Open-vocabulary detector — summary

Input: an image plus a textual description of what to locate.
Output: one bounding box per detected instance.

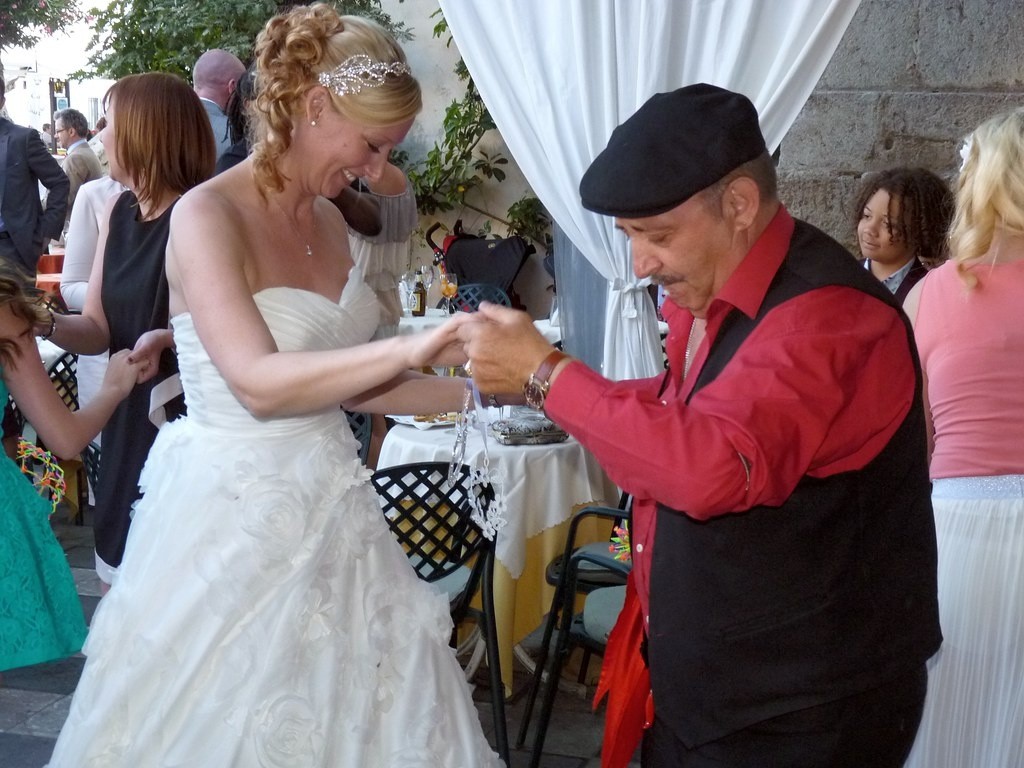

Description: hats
[580,83,768,219]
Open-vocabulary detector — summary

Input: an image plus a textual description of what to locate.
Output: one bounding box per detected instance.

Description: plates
[386,414,456,430]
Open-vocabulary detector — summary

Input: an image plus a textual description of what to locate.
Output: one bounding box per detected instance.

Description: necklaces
[269,189,316,256]
[683,318,696,379]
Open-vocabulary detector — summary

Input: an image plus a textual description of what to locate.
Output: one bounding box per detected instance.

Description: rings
[467,368,472,376]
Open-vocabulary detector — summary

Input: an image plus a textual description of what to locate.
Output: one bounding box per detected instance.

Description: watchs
[521,350,570,410]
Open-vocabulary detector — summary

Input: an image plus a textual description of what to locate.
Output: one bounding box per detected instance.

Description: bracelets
[41,310,56,340]
[462,359,472,370]
[489,393,501,408]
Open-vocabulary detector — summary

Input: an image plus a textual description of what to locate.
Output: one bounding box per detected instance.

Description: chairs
[435,284,511,314]
[366,463,511,768]
[16,352,103,527]
[514,491,632,768]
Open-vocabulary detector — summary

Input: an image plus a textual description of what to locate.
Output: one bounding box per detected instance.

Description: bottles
[412,272,426,316]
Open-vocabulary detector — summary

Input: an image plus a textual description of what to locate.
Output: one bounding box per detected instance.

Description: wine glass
[439,273,457,318]
[60,220,70,253]
[422,265,433,309]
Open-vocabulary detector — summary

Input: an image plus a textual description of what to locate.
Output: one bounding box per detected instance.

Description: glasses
[55,128,67,135]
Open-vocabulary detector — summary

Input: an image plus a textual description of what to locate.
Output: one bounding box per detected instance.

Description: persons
[50,0,512,768]
[900,107,1024,768]
[458,82,945,768]
[850,167,955,309]
[0,49,416,674]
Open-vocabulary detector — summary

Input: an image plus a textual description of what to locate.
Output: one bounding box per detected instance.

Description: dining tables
[399,308,470,376]
[375,408,633,698]
[8,336,110,506]
